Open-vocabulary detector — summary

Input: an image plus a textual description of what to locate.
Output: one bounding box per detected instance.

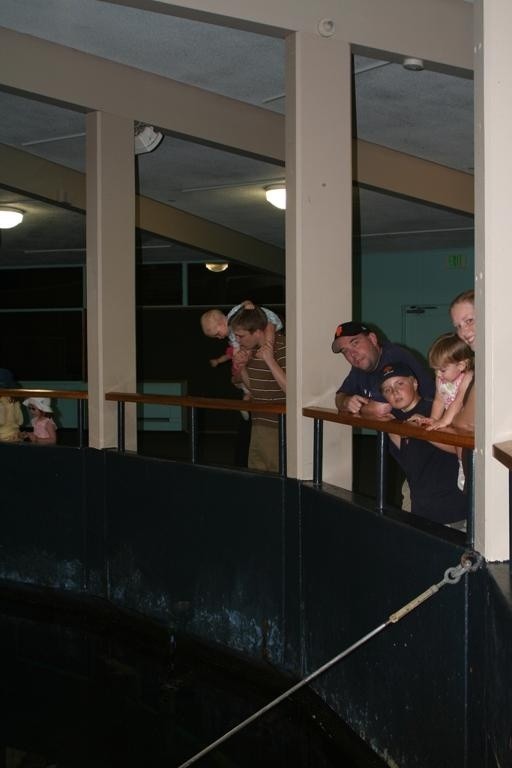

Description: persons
[200,300,283,368]
[448,288,474,351]
[404,332,474,431]
[230,306,286,472]
[331,321,436,509]
[22,397,58,444]
[377,363,468,533]
[0,368,26,443]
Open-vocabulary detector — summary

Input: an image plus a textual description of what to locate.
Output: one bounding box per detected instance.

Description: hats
[376,362,416,384]
[23,395,54,413]
[332,322,369,353]
